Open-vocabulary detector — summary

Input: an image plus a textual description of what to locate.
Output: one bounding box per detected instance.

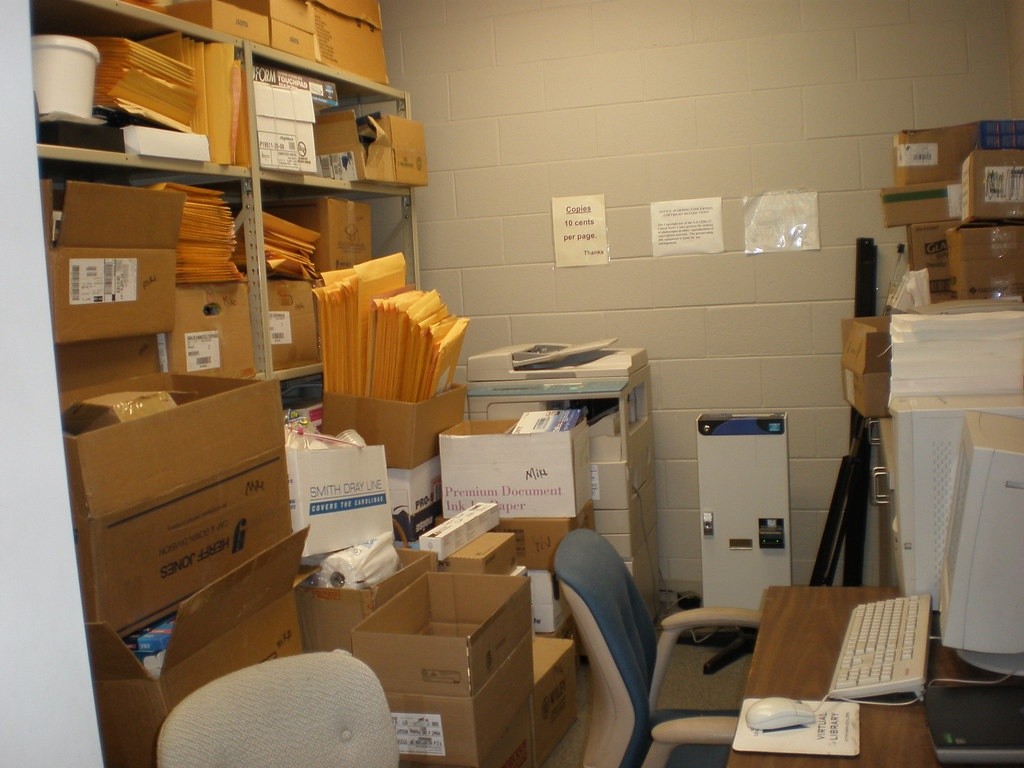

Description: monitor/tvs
[936,410,1024,677]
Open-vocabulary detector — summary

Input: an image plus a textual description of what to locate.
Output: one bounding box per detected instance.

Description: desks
[725,582,942,768]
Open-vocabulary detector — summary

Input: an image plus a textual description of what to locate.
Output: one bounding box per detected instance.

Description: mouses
[746,697,816,729]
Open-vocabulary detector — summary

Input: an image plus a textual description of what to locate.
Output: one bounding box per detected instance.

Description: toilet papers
[316,530,401,590]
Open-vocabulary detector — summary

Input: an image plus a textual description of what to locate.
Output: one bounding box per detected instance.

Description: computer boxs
[886,392,1024,611]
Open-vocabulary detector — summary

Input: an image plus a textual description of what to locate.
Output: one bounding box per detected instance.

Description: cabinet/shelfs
[30,0,421,382]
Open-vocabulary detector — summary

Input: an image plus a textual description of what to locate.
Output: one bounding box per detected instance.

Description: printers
[462,337,662,622]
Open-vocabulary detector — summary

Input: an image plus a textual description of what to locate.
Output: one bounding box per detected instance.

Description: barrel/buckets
[30,34,100,119]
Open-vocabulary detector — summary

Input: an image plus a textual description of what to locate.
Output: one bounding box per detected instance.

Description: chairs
[156,647,400,768]
[556,524,763,768]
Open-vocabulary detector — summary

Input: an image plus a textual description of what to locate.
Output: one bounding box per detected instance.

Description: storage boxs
[268,19,317,61]
[305,0,390,85]
[54,334,161,394]
[252,64,339,113]
[88,522,324,768]
[313,110,396,186]
[420,502,501,562]
[386,455,443,542]
[164,0,270,47]
[282,399,322,422]
[534,616,579,647]
[399,696,533,768]
[841,118,1024,417]
[252,81,317,174]
[437,416,591,519]
[527,569,571,634]
[321,381,468,470]
[167,280,320,377]
[352,570,535,768]
[226,195,373,279]
[311,419,322,433]
[293,547,439,658]
[375,114,429,188]
[434,498,596,571]
[529,633,579,768]
[439,531,517,576]
[57,372,293,637]
[223,0,313,34]
[36,179,187,344]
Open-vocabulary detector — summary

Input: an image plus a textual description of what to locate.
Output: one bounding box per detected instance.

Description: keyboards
[828,593,932,700]
